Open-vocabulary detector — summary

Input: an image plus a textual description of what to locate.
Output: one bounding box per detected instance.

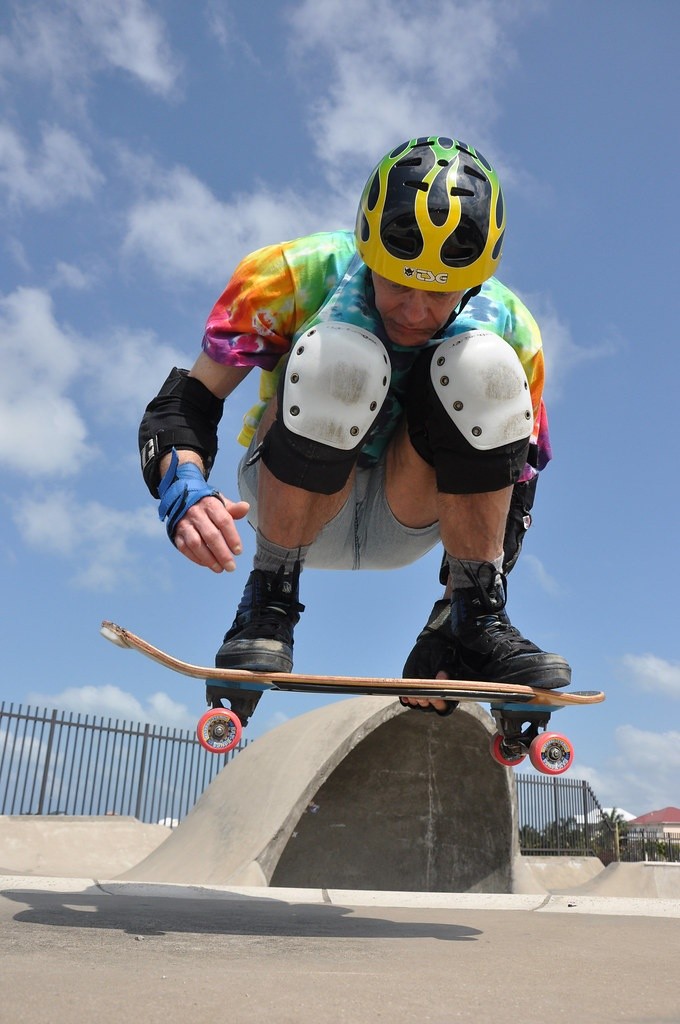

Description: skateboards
[101,620,607,774]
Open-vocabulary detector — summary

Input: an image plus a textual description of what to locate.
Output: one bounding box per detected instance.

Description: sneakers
[214,547,306,673]
[447,560,572,689]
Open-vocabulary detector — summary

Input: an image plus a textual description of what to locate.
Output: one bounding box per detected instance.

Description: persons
[138,136,571,715]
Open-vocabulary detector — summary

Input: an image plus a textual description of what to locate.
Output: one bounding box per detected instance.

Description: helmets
[354,136,506,293]
